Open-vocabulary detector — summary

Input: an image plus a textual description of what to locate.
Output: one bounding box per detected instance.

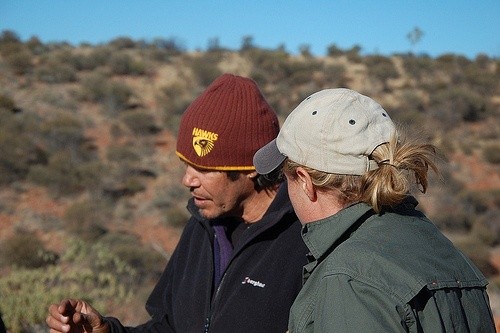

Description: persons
[42,72,307,333]
[250,86,498,333]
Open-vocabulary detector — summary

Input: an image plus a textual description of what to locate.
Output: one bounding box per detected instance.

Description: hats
[175,73,280,170]
[253,88,401,175]
